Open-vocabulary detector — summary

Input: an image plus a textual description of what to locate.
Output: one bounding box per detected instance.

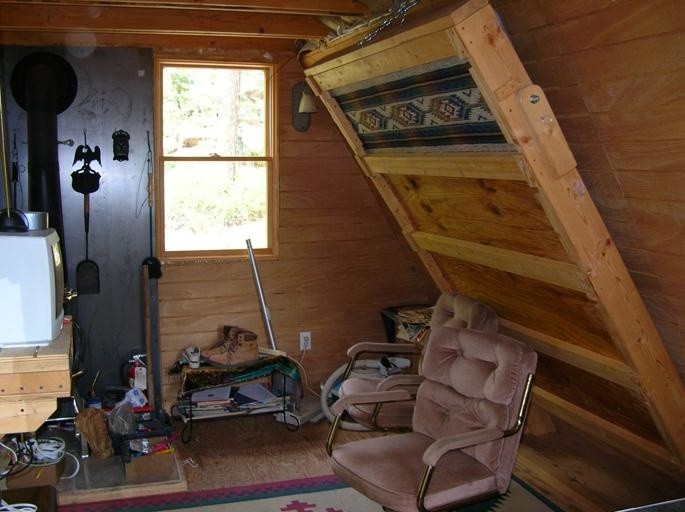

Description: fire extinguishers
[128,354,148,399]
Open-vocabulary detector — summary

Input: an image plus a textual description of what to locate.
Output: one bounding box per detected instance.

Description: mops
[243,237,336,426]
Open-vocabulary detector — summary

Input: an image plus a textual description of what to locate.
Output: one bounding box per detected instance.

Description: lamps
[292,79,319,133]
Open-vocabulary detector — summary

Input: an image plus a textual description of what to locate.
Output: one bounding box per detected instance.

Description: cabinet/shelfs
[172,354,302,443]
[0,314,76,436]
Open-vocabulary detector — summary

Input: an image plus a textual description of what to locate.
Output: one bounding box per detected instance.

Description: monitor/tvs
[1,231,64,346]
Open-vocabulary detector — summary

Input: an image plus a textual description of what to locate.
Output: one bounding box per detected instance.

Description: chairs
[321,294,499,457]
[326,326,538,511]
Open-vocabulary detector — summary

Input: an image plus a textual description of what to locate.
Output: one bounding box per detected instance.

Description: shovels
[75,193,99,295]
[142,151,161,278]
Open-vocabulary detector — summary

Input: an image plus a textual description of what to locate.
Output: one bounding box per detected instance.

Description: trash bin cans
[381,304,434,344]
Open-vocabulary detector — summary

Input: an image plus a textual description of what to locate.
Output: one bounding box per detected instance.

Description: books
[178,382,290,417]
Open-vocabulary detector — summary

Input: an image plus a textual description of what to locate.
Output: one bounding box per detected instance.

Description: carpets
[58,474,561,512]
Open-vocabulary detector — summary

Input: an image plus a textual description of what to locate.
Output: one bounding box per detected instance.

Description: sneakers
[183,325,259,369]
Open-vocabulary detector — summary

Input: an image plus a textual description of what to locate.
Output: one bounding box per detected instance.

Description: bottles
[90,399,101,409]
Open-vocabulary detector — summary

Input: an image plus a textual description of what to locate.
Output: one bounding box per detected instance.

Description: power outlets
[299,331,313,352]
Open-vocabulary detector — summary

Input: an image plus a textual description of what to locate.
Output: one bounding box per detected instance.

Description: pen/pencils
[36,462,47,479]
